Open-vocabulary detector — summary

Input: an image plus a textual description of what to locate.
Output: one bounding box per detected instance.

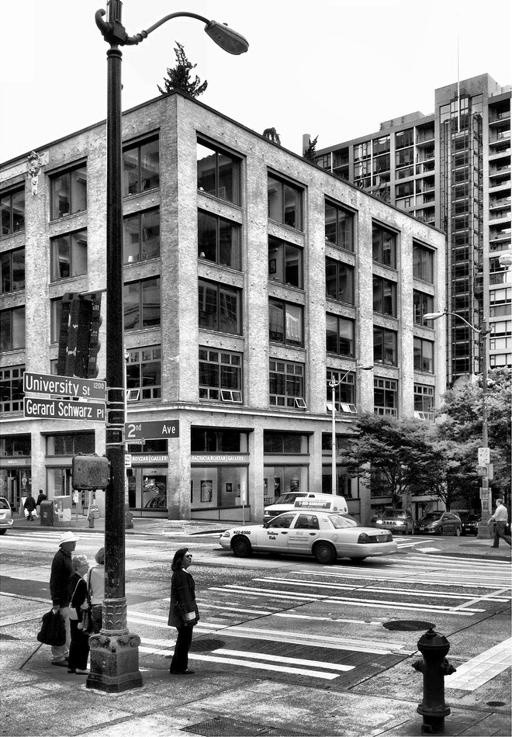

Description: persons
[36,489,49,507]
[487,498,511,549]
[164,545,203,675]
[41,532,104,679]
[22,494,36,521]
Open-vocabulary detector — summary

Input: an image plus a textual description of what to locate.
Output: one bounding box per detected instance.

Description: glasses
[184,553,193,558]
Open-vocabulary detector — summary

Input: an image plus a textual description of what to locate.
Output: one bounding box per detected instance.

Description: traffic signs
[121,418,181,441]
[21,371,104,399]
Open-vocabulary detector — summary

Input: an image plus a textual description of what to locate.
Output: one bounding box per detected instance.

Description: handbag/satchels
[82,601,103,635]
[69,606,79,621]
[37,606,68,645]
[173,602,193,620]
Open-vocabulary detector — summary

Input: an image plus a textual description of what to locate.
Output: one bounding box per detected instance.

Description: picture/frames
[200,480,212,502]
[226,483,232,492]
[290,480,299,492]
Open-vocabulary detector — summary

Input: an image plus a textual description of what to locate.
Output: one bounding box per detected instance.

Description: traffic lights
[25,398,105,422]
[95,311,105,375]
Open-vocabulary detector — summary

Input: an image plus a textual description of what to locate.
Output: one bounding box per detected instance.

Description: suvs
[0,495,17,536]
[262,491,349,530]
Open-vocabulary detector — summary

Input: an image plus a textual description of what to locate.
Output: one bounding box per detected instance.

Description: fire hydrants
[407,627,462,734]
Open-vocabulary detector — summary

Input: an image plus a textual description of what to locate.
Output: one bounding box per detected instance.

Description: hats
[59,529,83,546]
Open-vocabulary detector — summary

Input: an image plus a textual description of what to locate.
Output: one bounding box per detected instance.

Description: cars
[221,495,399,559]
[369,504,511,534]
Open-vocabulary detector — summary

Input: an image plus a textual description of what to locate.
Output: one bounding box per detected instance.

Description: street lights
[88,0,248,691]
[325,363,376,496]
[422,312,496,535]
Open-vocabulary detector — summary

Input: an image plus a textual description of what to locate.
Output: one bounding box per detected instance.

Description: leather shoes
[51,655,92,675]
[171,667,195,674]
